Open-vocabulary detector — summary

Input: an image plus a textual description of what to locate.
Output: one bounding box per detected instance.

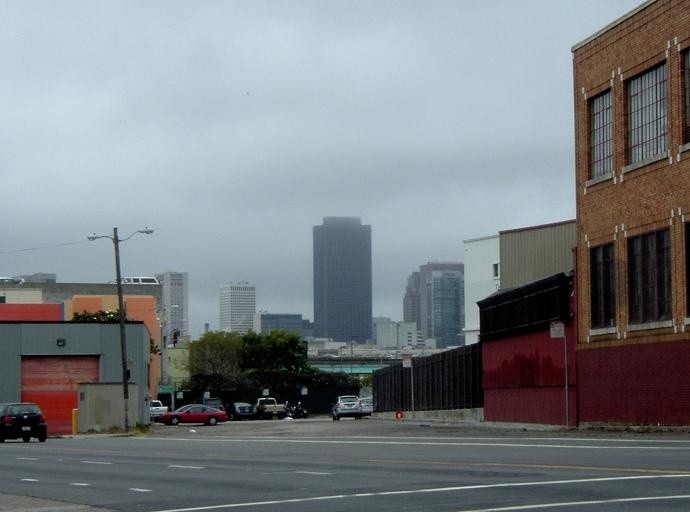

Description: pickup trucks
[252,397,285,419]
[150,400,169,421]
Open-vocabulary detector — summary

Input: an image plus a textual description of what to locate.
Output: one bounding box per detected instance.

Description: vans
[119,276,159,285]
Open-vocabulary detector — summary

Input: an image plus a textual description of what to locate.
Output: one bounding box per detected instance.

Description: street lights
[87,225,154,431]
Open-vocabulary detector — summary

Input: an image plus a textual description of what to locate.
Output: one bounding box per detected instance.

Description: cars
[332,395,363,421]
[229,402,256,420]
[160,404,228,426]
[0,403,47,444]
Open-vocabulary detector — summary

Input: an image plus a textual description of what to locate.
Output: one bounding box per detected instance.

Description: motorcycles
[286,402,308,420]
